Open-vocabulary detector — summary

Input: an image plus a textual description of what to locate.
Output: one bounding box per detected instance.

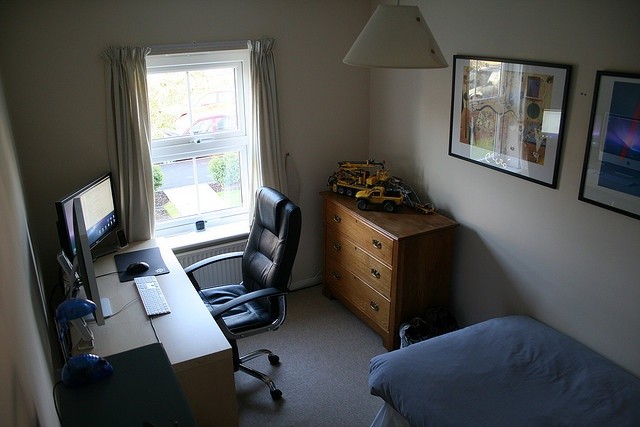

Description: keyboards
[133,276,171,316]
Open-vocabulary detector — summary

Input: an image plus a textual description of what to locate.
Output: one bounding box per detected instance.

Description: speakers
[57,252,78,282]
[71,317,94,349]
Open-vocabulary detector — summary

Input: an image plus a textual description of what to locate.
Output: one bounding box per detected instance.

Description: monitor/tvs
[67,199,112,326]
[54,172,119,262]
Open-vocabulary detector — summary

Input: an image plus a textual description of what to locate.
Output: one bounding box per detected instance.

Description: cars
[186,89,232,109]
[165,112,234,162]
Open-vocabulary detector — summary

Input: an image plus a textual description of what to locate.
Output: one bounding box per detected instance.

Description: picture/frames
[578,70,640,220]
[449,55,572,189]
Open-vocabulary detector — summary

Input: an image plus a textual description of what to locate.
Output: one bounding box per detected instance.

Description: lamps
[53,353,114,427]
[53,297,97,363]
[342,1,448,69]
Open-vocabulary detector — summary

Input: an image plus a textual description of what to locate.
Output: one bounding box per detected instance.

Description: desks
[65,238,239,427]
[55,343,199,427]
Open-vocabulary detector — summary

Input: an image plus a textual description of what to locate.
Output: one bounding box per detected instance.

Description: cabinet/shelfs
[319,190,460,352]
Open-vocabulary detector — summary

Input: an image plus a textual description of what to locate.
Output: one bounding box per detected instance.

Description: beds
[368,313,640,427]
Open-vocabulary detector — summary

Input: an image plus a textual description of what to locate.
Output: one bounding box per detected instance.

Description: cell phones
[117,229,127,247]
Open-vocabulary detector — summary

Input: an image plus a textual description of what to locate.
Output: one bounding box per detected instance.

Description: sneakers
[398,322,421,347]
[412,317,430,341]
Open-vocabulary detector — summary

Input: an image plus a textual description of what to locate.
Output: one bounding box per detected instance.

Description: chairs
[184,186,302,401]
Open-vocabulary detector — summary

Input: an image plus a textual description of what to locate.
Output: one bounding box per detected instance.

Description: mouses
[126,261,150,276]
